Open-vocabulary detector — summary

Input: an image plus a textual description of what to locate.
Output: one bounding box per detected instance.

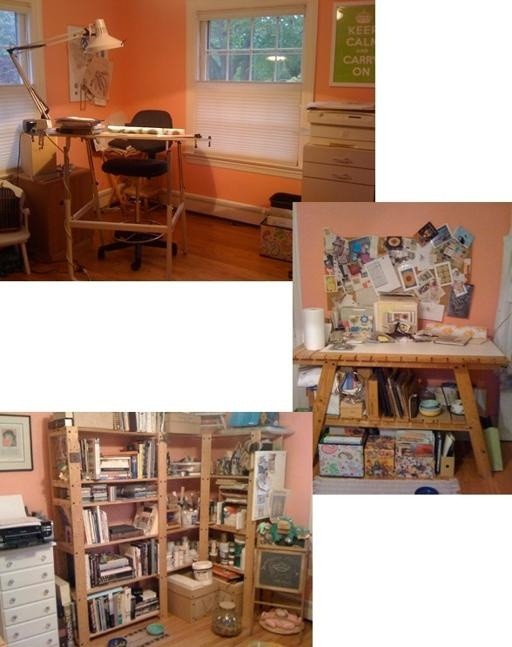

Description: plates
[108,638,126,647]
[147,624,165,635]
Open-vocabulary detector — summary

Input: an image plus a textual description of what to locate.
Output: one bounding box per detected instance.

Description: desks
[23,127,210,278]
[294,331,510,482]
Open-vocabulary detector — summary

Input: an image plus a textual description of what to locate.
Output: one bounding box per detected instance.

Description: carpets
[120,623,171,647]
[313,474,460,494]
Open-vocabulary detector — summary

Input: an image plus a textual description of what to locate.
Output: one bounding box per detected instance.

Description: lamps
[7,16,124,135]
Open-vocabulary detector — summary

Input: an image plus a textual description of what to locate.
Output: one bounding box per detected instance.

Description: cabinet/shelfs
[14,164,95,263]
[301,102,374,202]
[204,426,289,625]
[0,539,60,646]
[47,422,163,647]
[160,431,205,577]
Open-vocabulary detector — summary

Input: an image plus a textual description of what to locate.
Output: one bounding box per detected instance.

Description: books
[168,459,201,476]
[367,369,417,419]
[55,115,105,135]
[373,298,419,337]
[49,412,75,419]
[81,505,157,544]
[87,586,158,633]
[433,336,472,345]
[48,420,74,428]
[79,538,159,590]
[212,478,249,529]
[110,412,166,434]
[77,437,158,480]
[82,482,159,507]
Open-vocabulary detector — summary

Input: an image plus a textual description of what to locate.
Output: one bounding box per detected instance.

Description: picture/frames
[0,414,34,472]
[328,1,375,88]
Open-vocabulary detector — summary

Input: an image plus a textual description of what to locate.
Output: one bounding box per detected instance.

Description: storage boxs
[313,392,341,415]
[258,215,292,263]
[376,290,419,337]
[363,433,396,479]
[71,412,114,429]
[166,573,220,624]
[19,133,58,176]
[162,412,202,435]
[317,428,369,479]
[210,569,244,617]
[340,396,365,419]
[395,429,436,479]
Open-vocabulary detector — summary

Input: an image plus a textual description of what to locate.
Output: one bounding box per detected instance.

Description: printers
[0,495,55,551]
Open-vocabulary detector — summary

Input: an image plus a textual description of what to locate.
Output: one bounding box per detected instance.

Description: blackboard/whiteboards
[258,551,302,590]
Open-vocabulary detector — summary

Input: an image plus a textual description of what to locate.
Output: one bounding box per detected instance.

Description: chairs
[0,180,34,279]
[97,109,179,272]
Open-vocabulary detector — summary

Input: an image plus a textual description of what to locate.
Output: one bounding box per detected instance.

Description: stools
[121,184,162,215]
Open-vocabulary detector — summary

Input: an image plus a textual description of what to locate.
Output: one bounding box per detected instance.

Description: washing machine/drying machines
[302,306,331,351]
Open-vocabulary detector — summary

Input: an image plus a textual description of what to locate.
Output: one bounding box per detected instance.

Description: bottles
[212,602,241,637]
